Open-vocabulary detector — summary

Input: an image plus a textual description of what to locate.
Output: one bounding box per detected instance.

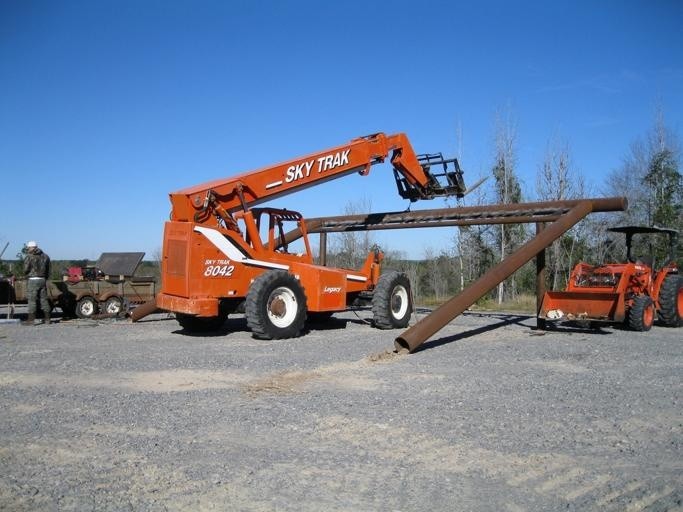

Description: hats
[27,242,37,246]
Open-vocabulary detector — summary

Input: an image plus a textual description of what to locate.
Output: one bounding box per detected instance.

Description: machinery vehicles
[538,226,683,331]
[0,253,156,318]
[155,132,487,340]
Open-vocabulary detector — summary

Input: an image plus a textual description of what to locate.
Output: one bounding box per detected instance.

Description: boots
[42,313,50,324]
[22,313,35,326]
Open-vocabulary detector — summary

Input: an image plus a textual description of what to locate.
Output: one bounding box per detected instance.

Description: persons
[21,242,51,325]
